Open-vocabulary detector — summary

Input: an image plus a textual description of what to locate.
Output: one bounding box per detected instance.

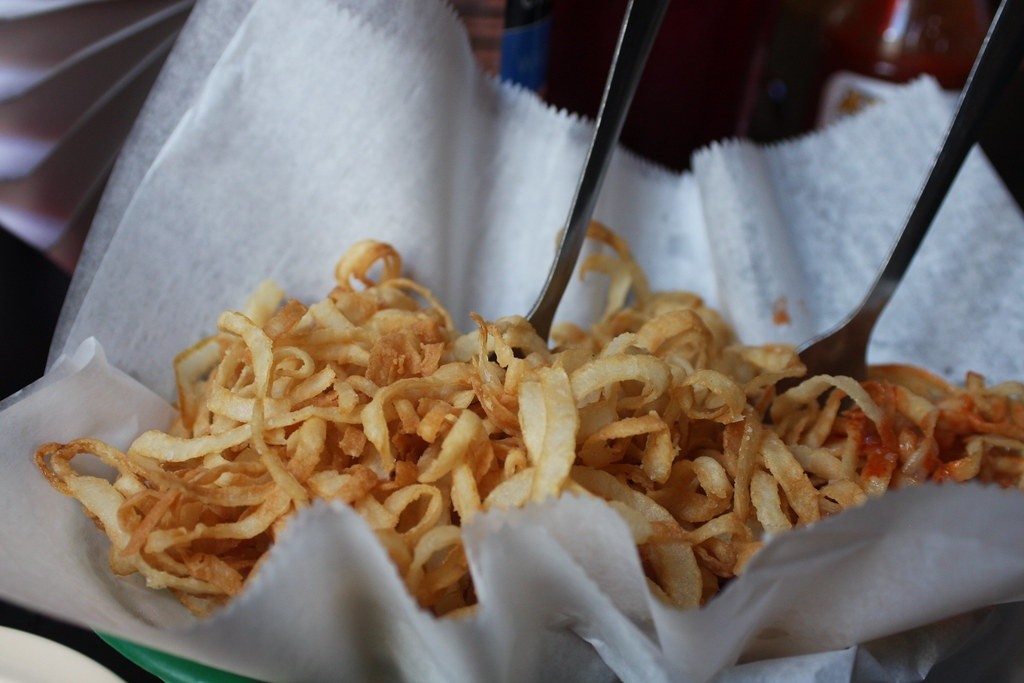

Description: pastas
[32,219,1024,617]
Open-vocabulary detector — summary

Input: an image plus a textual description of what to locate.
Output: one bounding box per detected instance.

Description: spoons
[750,0,1024,434]
[480,3,671,376]
